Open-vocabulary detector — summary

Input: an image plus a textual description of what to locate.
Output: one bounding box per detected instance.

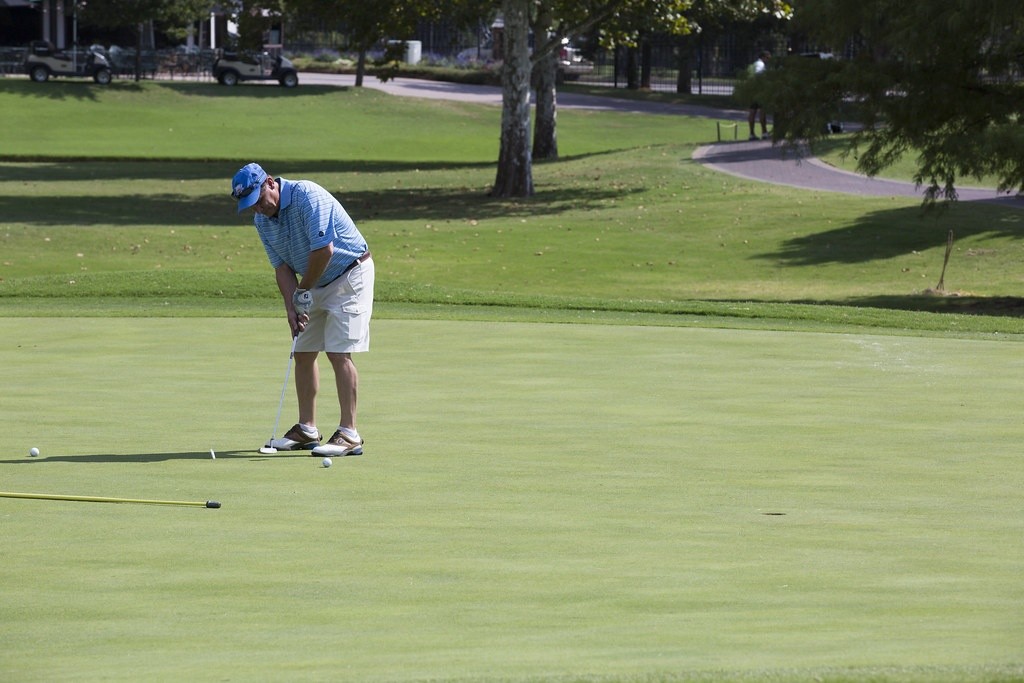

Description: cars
[488,33,594,84]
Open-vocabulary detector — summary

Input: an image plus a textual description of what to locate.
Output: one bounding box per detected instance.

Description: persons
[231,162,375,456]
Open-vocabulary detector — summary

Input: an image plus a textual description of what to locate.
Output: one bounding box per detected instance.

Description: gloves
[292,286,314,316]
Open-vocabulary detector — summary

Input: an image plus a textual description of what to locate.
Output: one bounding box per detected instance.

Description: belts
[320,249,371,288]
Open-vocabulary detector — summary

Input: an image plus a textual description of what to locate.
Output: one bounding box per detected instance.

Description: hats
[232,162,268,214]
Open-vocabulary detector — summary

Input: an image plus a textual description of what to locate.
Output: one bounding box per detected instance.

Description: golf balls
[323,458,332,467]
[30,447,39,456]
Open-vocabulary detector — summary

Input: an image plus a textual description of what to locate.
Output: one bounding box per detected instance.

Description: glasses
[230,172,265,203]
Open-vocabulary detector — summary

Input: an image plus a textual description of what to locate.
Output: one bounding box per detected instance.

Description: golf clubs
[259,314,300,454]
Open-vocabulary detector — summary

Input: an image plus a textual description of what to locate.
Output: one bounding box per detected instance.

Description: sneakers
[266,423,322,451]
[311,429,364,457]
